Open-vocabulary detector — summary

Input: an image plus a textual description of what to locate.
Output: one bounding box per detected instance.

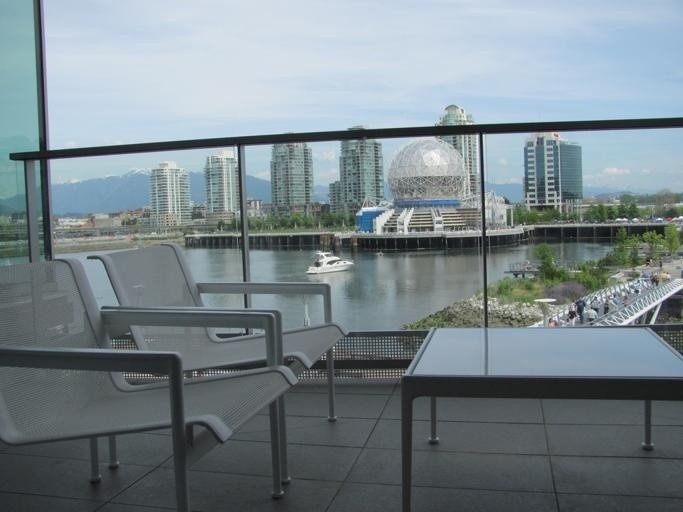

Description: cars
[612,216,683,224]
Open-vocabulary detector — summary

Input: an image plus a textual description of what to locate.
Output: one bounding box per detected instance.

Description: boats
[305,249,354,275]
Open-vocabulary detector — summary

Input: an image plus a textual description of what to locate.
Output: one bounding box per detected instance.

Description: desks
[402,326,683,511]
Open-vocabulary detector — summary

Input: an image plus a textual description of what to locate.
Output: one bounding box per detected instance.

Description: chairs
[0,259,286,512]
[87,244,346,485]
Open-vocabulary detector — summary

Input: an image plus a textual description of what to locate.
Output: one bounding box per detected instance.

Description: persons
[547,256,670,328]
